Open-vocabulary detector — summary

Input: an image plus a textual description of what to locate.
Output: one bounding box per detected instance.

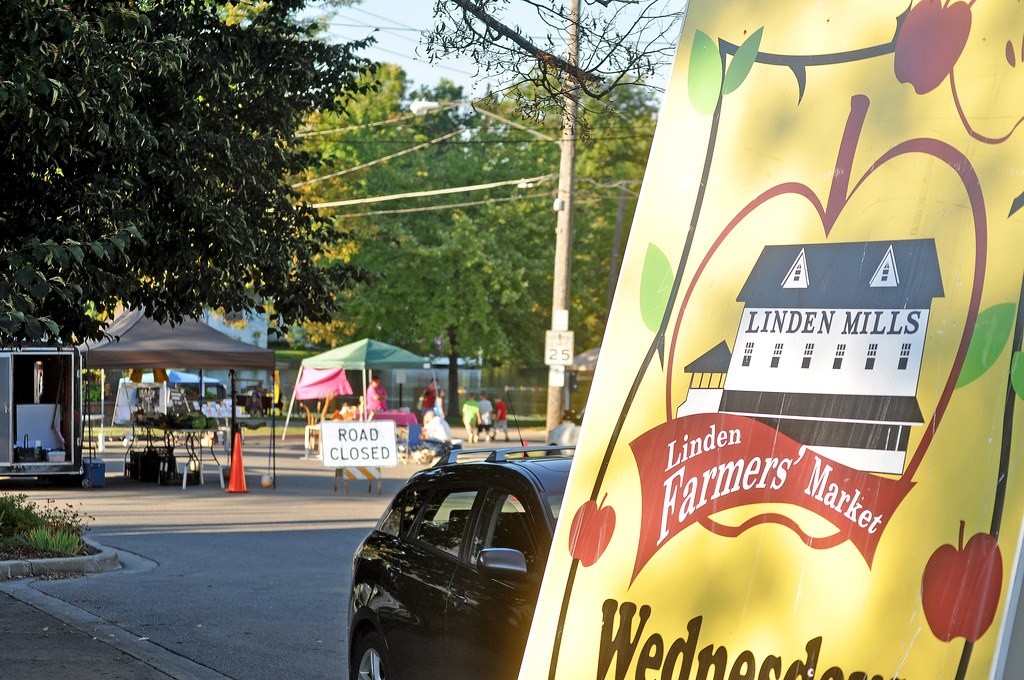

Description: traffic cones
[225,432,249,493]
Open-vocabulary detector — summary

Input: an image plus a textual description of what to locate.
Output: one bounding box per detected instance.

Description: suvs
[347,446,578,680]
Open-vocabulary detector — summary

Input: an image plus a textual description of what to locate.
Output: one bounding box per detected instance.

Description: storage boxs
[47,452,66,462]
[125,446,200,486]
[82,457,105,487]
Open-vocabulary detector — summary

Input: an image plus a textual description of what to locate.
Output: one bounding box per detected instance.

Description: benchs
[447,509,537,555]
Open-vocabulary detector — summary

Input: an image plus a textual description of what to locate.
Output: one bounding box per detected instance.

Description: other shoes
[485,435,496,443]
[469,434,479,443]
[505,436,510,442]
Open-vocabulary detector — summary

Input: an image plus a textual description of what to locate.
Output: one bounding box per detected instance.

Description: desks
[356,411,418,442]
[304,425,322,461]
[122,424,225,490]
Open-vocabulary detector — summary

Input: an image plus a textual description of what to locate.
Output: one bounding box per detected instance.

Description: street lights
[408,0,582,456]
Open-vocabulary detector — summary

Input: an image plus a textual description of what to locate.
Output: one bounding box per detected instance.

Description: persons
[419,380,452,443]
[462,391,509,443]
[365,376,388,413]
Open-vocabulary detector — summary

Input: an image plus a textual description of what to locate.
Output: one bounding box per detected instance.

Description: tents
[283,338,438,441]
[75,300,277,491]
[565,348,600,410]
